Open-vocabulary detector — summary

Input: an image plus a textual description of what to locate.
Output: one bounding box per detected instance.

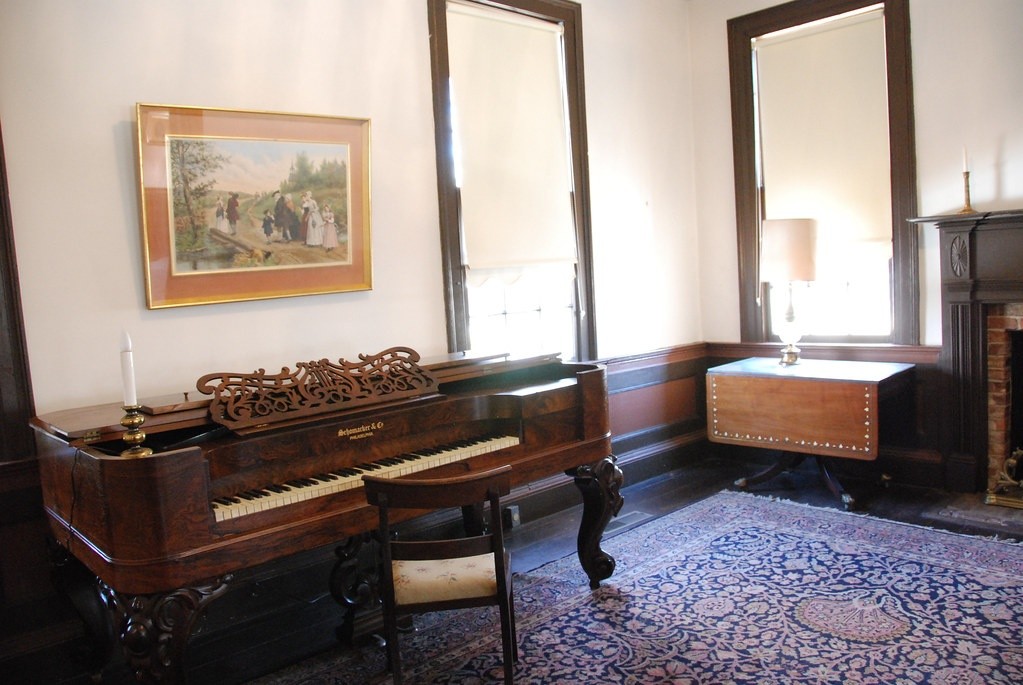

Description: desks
[706,354,917,511]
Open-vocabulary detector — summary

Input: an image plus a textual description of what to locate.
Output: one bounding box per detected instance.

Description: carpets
[244,487,1023,685]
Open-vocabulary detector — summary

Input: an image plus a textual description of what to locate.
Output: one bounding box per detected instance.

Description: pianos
[23,346,623,680]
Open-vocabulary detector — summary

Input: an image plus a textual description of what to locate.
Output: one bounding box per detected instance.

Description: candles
[119,330,138,407]
[961,143,968,171]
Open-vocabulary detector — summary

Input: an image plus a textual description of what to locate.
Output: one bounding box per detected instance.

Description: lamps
[758,218,818,366]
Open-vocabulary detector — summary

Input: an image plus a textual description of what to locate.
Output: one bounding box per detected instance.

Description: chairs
[361,463,519,685]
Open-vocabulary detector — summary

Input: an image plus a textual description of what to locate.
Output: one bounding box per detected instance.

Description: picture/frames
[130,102,374,310]
